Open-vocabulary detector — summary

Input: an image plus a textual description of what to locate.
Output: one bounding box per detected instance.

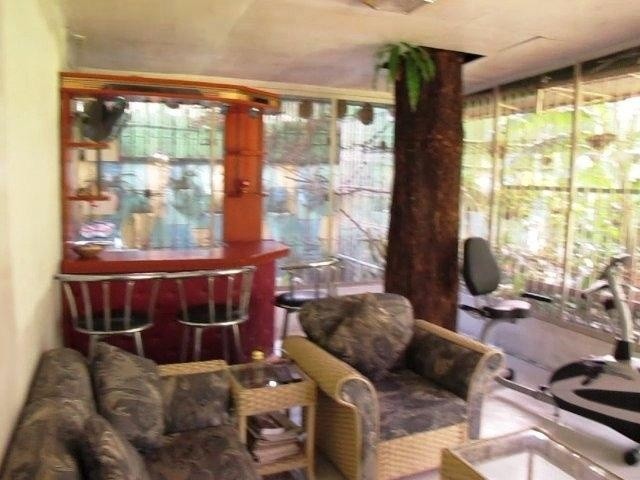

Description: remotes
[284,364,304,380]
[273,367,288,383]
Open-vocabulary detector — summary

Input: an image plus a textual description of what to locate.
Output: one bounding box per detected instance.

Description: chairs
[53,271,165,358]
[268,255,339,342]
[282,319,506,480]
[165,265,257,366]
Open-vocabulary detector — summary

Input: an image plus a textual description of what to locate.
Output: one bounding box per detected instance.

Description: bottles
[251,350,264,385]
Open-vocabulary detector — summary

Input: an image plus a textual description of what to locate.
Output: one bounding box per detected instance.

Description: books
[246,410,304,464]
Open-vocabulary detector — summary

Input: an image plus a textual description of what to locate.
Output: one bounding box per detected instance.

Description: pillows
[0,342,232,479]
[299,293,414,383]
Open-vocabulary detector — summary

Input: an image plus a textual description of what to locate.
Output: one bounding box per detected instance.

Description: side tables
[228,358,316,480]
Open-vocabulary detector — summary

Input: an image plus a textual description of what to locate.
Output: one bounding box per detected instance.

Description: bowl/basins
[71,245,105,257]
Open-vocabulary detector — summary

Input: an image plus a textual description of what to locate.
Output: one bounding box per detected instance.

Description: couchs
[1,349,262,480]
